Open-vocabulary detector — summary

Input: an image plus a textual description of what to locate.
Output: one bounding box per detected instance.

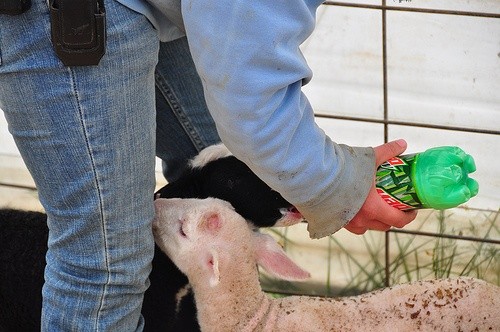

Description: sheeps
[151,197,499,332]
[0,143,306,332]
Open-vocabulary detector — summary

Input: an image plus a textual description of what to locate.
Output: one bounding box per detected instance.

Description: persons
[0,0,422,332]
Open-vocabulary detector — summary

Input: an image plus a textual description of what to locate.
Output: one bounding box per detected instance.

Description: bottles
[375,146,479,212]
